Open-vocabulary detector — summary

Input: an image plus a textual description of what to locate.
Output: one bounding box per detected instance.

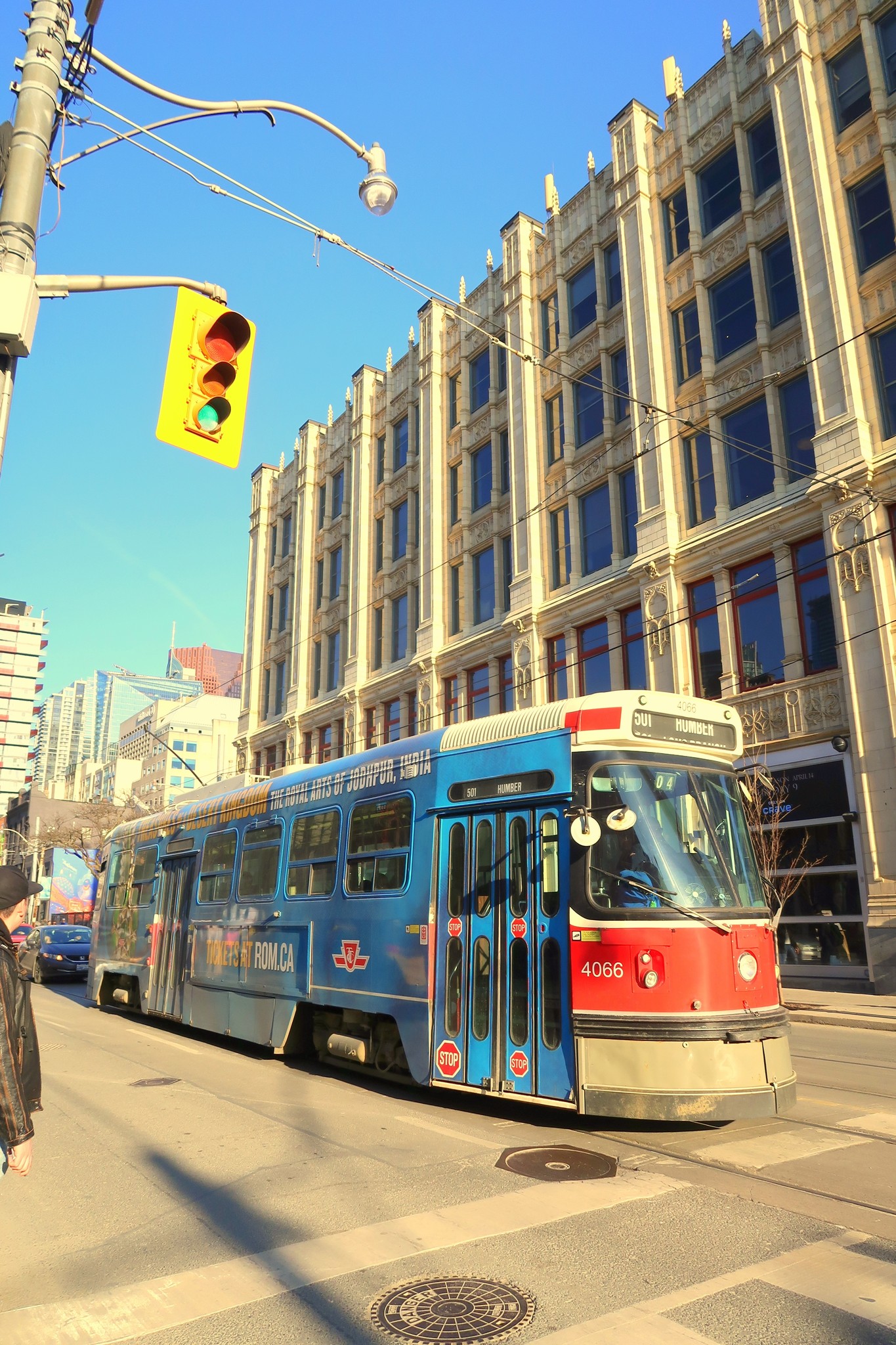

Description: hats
[0,865,43,909]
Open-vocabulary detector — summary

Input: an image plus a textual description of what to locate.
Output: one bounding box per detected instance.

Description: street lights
[1,828,39,924]
[2,0,399,480]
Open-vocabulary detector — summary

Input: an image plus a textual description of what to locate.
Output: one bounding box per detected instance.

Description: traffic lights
[156,286,256,470]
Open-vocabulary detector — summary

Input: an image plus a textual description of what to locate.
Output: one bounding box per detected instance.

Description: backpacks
[617,870,660,908]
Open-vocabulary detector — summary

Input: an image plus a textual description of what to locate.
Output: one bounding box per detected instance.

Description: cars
[10,924,34,949]
[17,924,92,984]
[785,931,833,963]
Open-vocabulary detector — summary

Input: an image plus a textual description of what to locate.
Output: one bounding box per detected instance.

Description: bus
[89,690,797,1121]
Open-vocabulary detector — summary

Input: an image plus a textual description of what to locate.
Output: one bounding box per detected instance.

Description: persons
[69,932,82,942]
[624,817,672,877]
[45,931,58,943]
[0,866,43,1176]
[779,924,800,965]
[40,917,47,924]
[59,918,68,925]
[47,920,57,925]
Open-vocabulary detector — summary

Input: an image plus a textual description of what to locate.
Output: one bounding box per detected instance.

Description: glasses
[649,828,663,833]
